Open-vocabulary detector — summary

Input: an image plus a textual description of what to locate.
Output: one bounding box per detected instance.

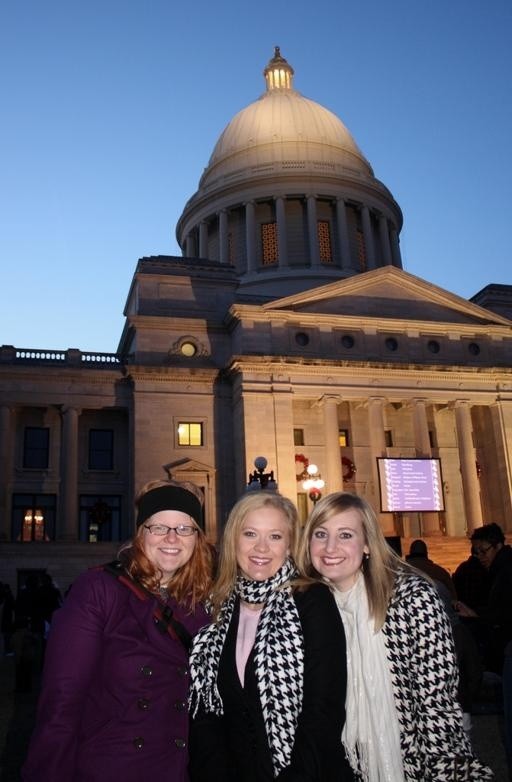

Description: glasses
[144,525,198,536]
[471,544,493,555]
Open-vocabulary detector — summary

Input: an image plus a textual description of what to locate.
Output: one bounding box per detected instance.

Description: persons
[17,480,211,781]
[189,491,356,781]
[404,522,512,782]
[0,568,72,673]
[294,489,495,782]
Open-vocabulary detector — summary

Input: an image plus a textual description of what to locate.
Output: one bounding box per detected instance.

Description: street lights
[302,463,325,508]
[248,456,275,486]
